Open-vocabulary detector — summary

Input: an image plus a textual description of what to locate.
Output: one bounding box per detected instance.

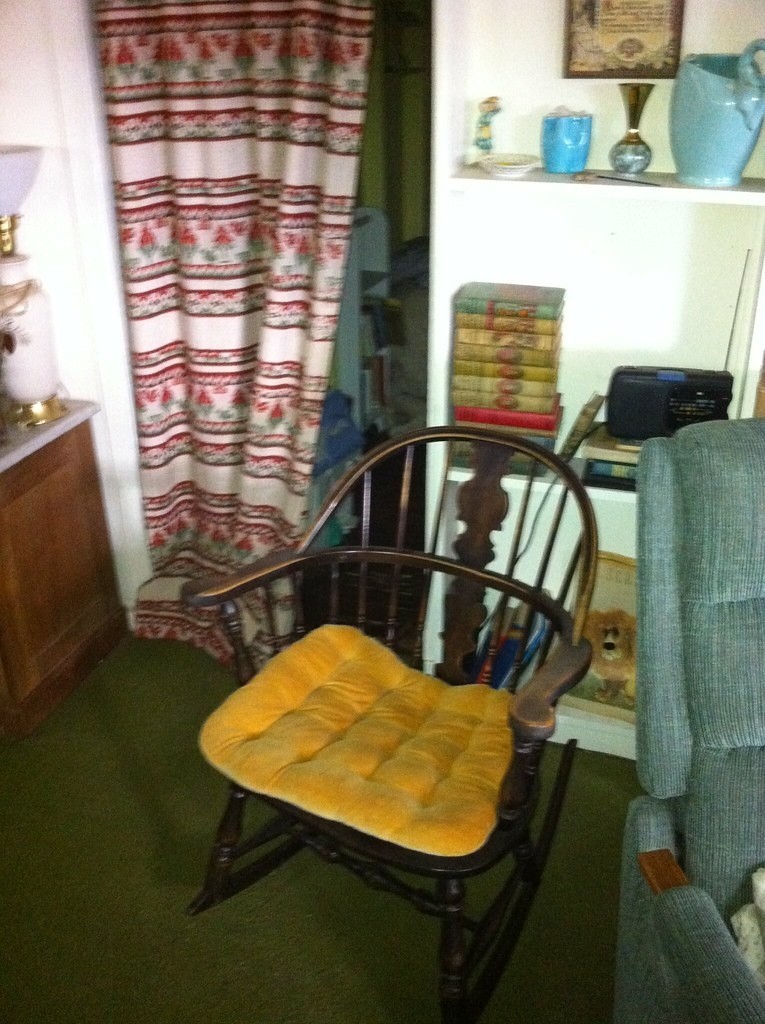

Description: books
[558,391,605,466]
[582,418,642,479]
[449,282,566,479]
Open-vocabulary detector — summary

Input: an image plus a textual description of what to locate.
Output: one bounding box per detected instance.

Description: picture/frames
[559,549,639,721]
[563,0,686,79]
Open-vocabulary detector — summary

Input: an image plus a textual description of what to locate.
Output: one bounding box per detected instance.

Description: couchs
[611,413,765,1023]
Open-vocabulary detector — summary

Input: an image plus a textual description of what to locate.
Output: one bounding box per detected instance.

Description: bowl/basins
[477,152,539,179]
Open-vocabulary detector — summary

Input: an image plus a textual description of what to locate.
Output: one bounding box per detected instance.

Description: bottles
[607,82,655,172]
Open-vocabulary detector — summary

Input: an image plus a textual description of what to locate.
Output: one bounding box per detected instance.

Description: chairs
[183,429,606,1023]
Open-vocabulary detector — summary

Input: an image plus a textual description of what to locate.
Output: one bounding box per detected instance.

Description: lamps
[0,143,67,430]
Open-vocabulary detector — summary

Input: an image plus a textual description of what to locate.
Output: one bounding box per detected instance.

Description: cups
[540,115,593,173]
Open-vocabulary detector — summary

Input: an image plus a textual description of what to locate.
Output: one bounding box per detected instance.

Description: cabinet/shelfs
[423,0,765,763]
[0,419,127,740]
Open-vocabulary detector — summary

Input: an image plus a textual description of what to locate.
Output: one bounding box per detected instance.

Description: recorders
[606,365,735,441]
